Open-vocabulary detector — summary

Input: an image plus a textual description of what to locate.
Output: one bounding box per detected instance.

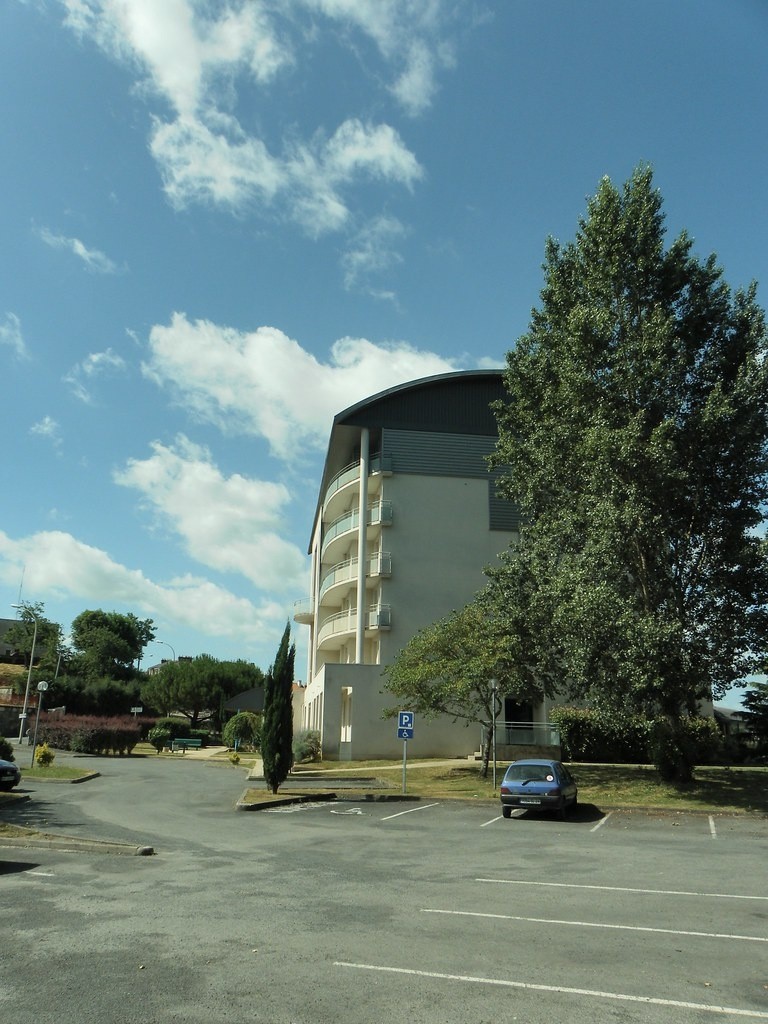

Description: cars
[500,759,577,818]
[0,759,21,792]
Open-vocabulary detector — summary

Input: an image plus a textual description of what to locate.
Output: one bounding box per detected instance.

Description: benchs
[175,738,201,750]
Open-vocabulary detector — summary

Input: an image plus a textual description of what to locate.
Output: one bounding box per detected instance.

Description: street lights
[156,640,176,662]
[488,678,501,790]
[10,604,37,744]
[30,681,48,768]
